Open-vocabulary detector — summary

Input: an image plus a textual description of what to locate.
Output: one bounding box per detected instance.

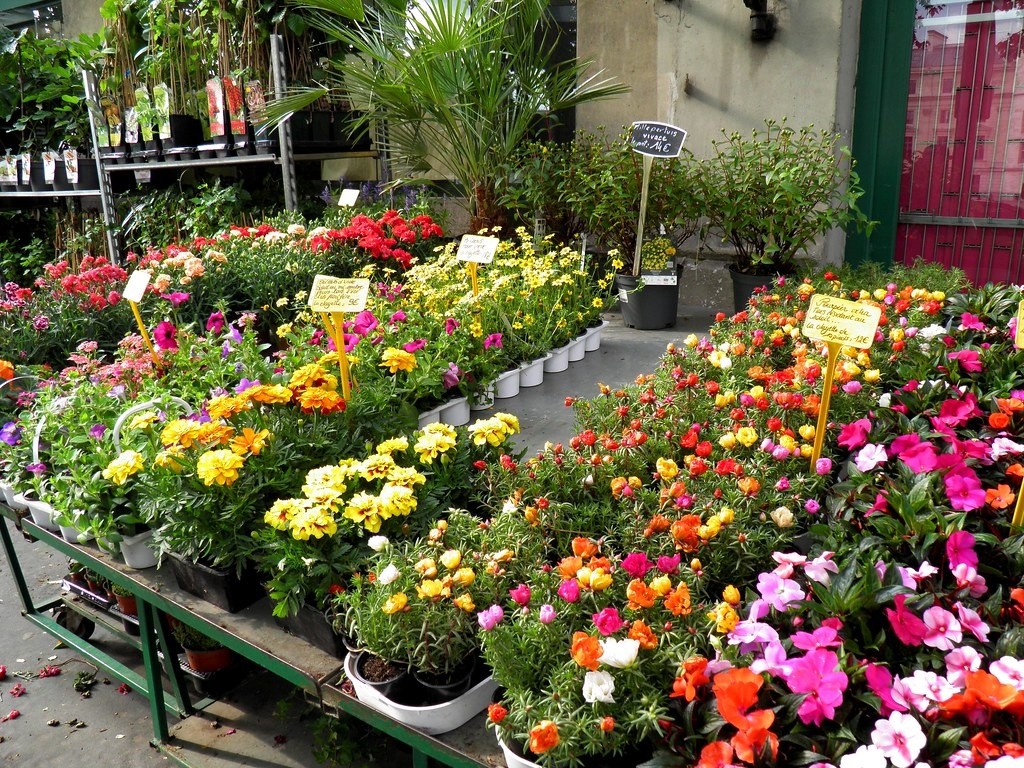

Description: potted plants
[687,116,882,316]
[0,0,410,192]
[555,124,725,334]
[502,129,637,299]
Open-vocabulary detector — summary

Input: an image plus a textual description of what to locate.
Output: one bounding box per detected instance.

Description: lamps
[744,0,777,42]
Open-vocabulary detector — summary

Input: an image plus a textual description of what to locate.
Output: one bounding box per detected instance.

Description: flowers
[0,209,1024,768]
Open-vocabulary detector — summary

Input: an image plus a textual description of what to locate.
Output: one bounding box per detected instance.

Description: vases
[491,686,515,711]
[12,492,60,532]
[0,479,28,510]
[411,652,476,705]
[95,537,122,554]
[494,725,542,768]
[354,650,423,707]
[344,651,500,736]
[160,541,272,614]
[473,352,554,411]
[542,320,610,372]
[119,529,167,569]
[591,734,657,768]
[53,516,93,543]
[536,752,594,768]
[342,634,361,658]
[417,391,479,431]
[270,595,346,658]
